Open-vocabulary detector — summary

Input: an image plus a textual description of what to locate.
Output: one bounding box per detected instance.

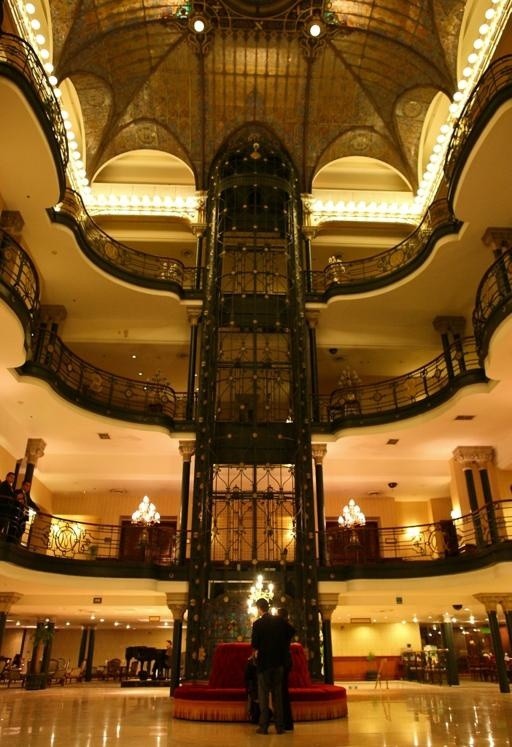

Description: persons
[250,598,285,734]
[0,471,24,541]
[9,481,42,543]
[277,608,296,734]
[152,639,172,680]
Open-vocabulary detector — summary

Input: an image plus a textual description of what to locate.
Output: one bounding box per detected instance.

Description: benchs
[170,640,348,721]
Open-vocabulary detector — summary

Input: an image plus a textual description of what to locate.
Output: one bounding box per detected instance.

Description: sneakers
[255,727,268,734]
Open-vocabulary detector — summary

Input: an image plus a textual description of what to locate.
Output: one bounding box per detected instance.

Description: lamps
[306,14,323,36]
[245,574,278,629]
[131,494,161,544]
[337,497,366,548]
[190,11,208,35]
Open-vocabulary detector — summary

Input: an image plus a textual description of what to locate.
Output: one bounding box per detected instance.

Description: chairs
[466,654,497,682]
[165,650,187,680]
[0,656,87,688]
